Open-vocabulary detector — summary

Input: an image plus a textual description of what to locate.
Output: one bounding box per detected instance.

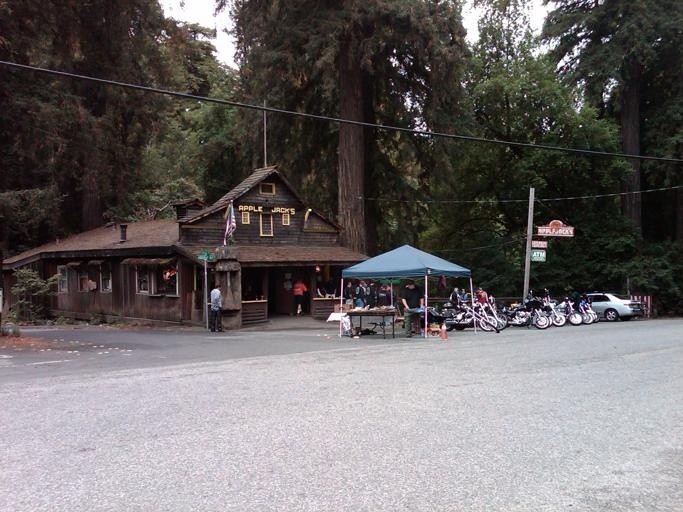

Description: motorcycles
[440,287,598,332]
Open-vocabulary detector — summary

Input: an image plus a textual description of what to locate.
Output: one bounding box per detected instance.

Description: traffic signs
[197,255,206,260]
[207,254,217,261]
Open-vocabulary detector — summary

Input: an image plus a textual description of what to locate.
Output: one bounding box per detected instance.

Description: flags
[223,204,237,247]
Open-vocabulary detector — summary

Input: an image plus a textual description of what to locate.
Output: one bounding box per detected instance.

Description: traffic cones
[440,320,447,339]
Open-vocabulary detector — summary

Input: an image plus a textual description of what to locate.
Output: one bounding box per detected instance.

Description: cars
[585,292,643,322]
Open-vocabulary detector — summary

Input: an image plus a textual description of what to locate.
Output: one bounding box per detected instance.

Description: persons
[341,274,593,336]
[210,283,226,332]
[292,277,308,319]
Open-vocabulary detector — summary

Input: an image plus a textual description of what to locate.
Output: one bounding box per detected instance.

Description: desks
[347,307,397,340]
